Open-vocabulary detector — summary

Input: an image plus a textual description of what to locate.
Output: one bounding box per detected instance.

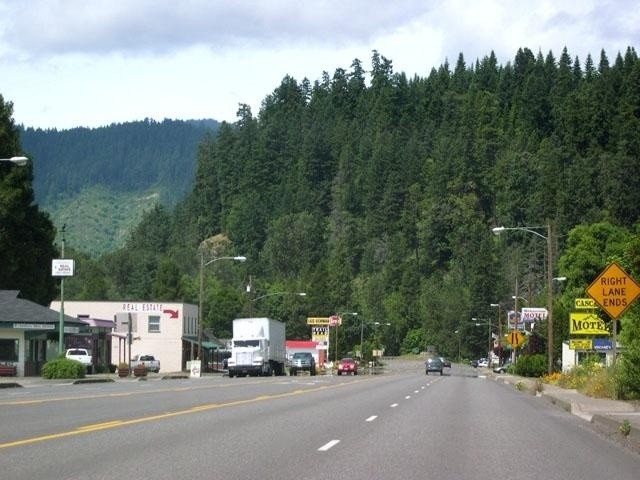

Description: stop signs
[330,315,342,327]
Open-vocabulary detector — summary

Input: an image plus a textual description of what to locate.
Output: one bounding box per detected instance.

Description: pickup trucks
[65,347,94,375]
[131,354,160,373]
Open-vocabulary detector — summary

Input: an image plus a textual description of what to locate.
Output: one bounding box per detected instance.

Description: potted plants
[134,364,149,376]
[118,362,131,377]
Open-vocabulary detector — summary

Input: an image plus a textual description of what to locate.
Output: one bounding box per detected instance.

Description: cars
[438,356,451,368]
[492,361,511,374]
[425,358,443,376]
[337,358,358,375]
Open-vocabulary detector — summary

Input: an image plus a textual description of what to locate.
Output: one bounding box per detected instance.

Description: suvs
[289,352,316,376]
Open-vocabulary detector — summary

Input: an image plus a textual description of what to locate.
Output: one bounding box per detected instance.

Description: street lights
[490,303,502,366]
[197,256,247,360]
[247,275,307,317]
[335,312,358,367]
[454,330,461,366]
[374,323,391,363]
[359,320,380,361]
[472,317,491,366]
[491,223,553,375]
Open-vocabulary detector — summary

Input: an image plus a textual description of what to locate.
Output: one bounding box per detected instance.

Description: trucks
[227,317,286,377]
